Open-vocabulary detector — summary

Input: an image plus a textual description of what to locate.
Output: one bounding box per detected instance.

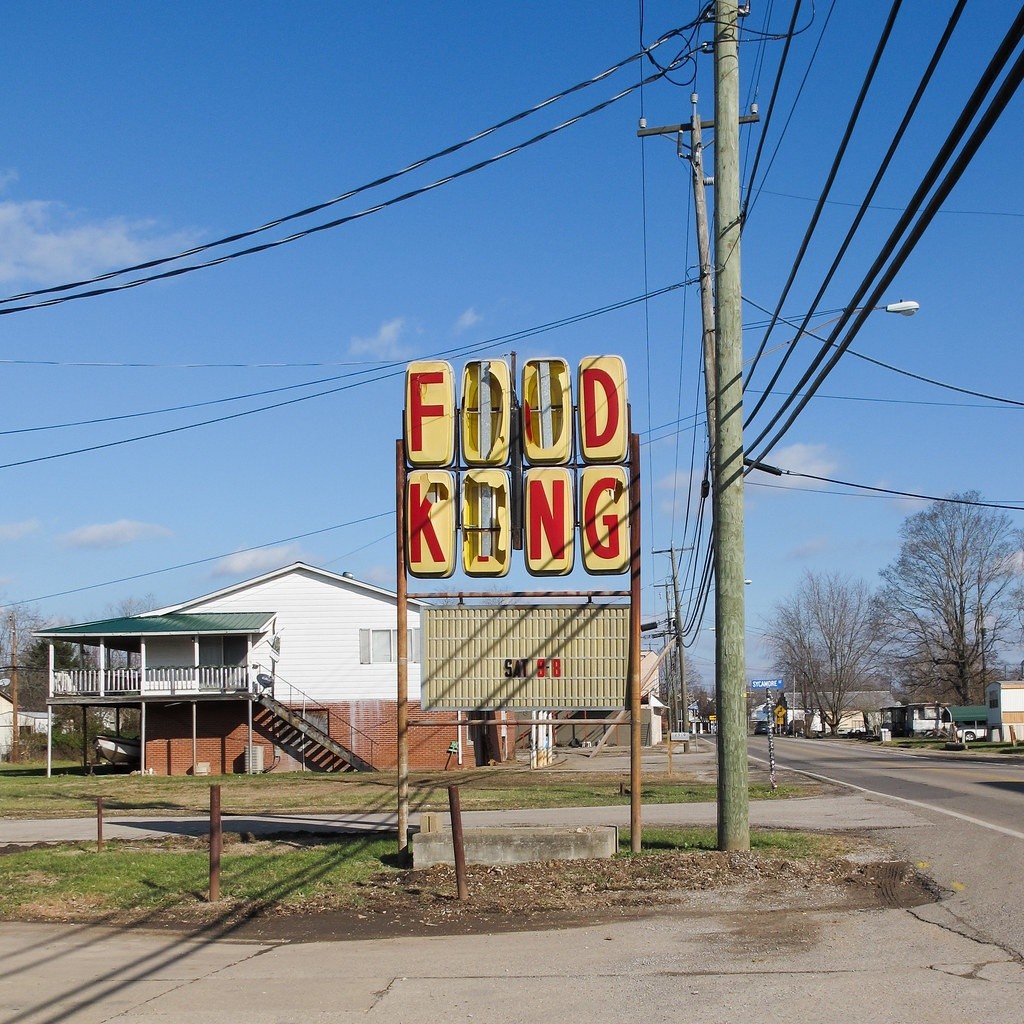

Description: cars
[956,720,988,742]
[850,723,875,735]
[754,721,768,735]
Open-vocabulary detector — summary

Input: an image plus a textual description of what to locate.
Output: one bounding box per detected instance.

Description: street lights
[703,302,917,496]
[673,580,754,753]
